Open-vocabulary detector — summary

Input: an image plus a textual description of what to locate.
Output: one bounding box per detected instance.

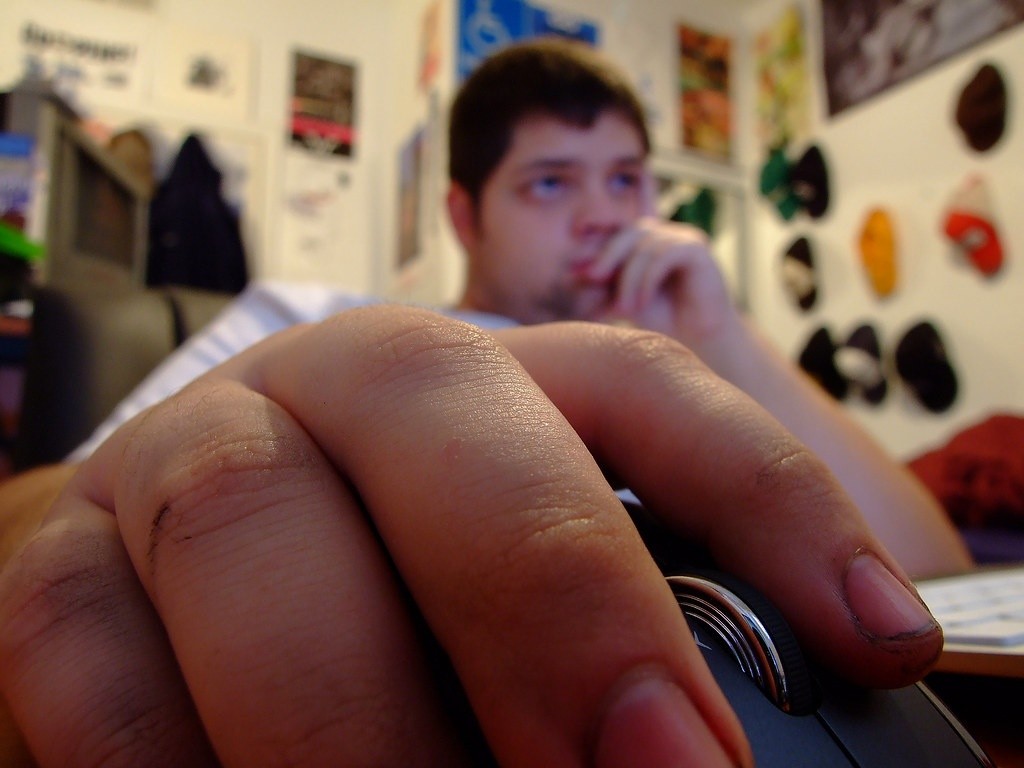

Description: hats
[780,238,817,308]
[858,209,899,296]
[934,163,1006,274]
[893,321,958,413]
[669,187,715,236]
[759,148,801,220]
[791,146,828,217]
[833,323,888,404]
[797,328,852,400]
[956,63,1008,152]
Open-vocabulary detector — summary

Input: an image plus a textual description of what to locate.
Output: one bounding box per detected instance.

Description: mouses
[384,494,995,768]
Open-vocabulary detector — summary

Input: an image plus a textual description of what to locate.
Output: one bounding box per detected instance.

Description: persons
[0,37,973,768]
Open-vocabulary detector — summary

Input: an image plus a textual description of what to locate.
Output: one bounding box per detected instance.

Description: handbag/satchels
[145,133,250,293]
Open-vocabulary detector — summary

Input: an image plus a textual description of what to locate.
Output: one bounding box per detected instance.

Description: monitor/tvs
[27,108,146,300]
